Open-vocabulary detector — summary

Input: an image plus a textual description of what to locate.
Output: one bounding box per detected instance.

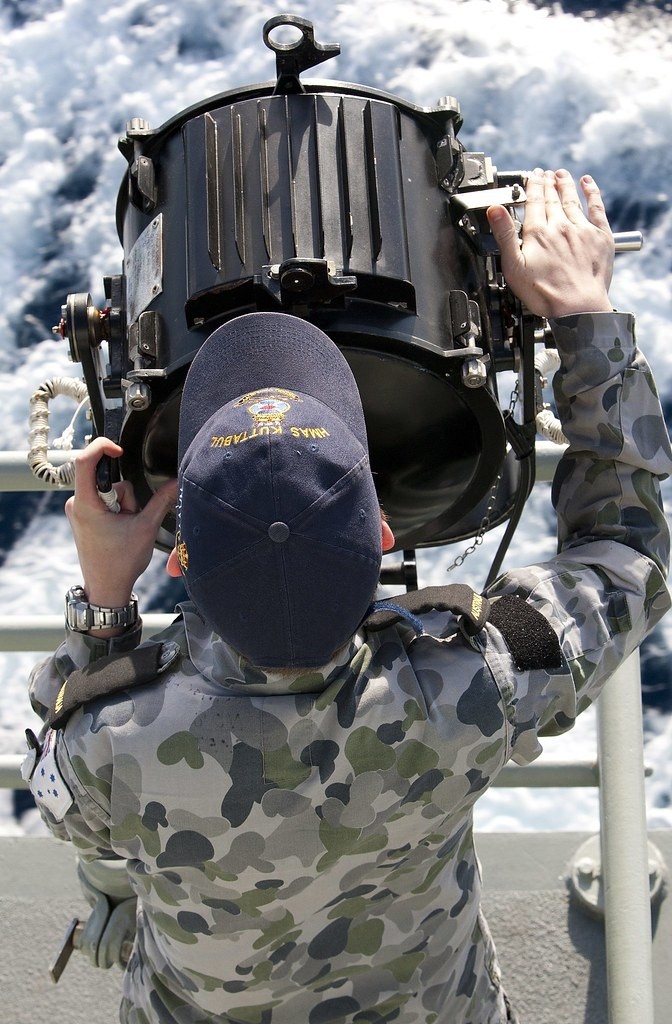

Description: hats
[174,311,384,667]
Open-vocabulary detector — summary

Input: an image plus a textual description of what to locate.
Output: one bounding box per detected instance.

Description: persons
[21,167,672,1024]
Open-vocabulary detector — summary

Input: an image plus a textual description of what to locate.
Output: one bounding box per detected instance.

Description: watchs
[63,584,143,637]
